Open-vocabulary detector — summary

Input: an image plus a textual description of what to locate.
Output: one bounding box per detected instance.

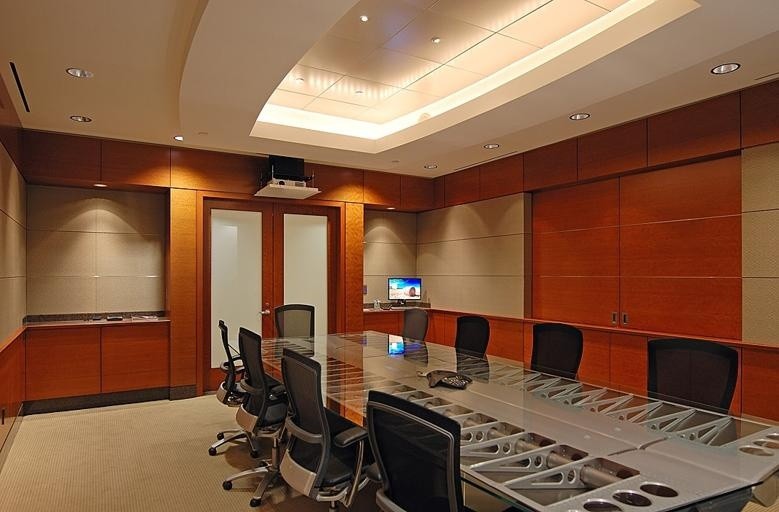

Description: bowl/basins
[381,305,392,309]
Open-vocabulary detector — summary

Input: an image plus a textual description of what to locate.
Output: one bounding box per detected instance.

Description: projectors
[267,178,307,187]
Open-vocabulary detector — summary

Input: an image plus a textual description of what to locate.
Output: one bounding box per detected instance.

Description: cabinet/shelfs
[25,318,172,398]
[363,307,428,335]
[531,156,739,338]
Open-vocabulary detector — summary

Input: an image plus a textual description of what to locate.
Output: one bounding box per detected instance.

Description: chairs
[278,347,370,511]
[220,326,285,506]
[365,389,477,511]
[455,314,490,354]
[274,303,315,338]
[646,338,740,410]
[531,324,582,376]
[210,320,261,457]
[403,308,427,340]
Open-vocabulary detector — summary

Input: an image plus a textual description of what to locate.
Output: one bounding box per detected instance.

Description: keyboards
[390,306,420,310]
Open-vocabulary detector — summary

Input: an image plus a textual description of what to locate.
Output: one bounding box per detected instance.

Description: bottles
[373,298,380,309]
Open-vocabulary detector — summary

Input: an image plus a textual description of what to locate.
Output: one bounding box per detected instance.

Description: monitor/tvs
[388,334,406,354]
[387,277,422,304]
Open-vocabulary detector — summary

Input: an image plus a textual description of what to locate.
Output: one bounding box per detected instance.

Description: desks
[228,330,778,509]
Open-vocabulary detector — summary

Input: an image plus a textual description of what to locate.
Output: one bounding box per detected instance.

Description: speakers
[269,155,304,180]
